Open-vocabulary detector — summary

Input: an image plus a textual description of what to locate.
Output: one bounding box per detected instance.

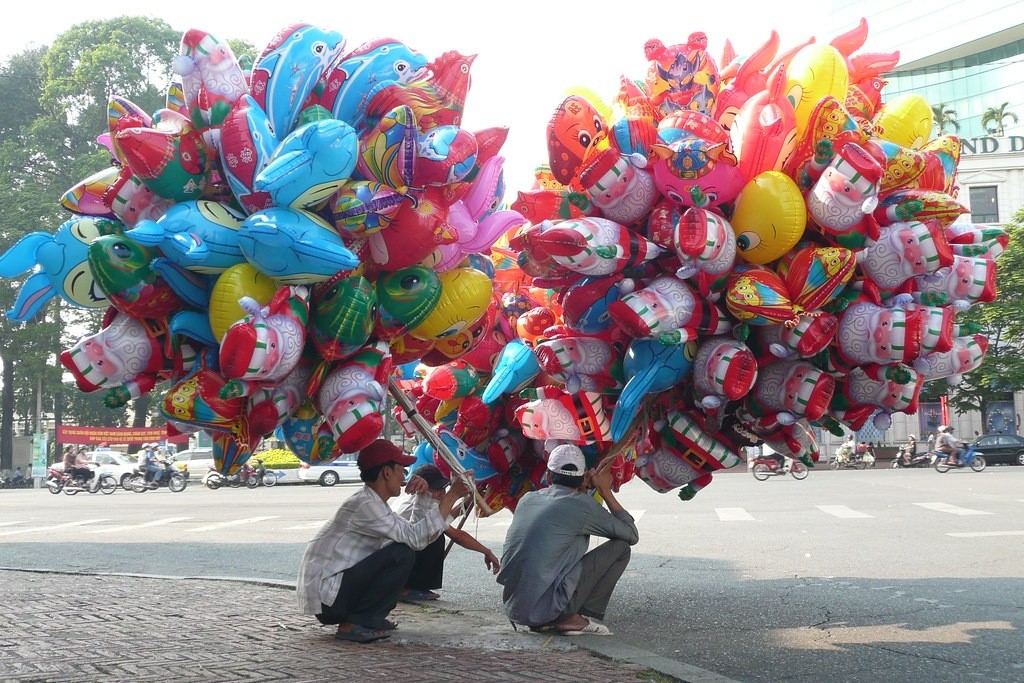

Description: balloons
[0,17,1009,515]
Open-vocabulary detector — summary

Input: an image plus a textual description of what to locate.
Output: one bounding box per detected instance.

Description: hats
[548,444,586,477]
[357,440,417,467]
[413,465,451,489]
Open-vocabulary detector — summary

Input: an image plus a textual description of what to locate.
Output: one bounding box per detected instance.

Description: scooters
[890,444,932,468]
[120,450,188,493]
[204,456,277,489]
[46,461,118,495]
[827,444,877,471]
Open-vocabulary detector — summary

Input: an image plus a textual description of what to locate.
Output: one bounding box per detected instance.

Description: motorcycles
[929,441,987,473]
[748,450,809,482]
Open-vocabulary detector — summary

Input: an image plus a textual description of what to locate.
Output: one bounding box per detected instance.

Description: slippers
[563,616,614,636]
[335,625,383,643]
[531,624,555,632]
[400,590,439,601]
[361,619,397,630]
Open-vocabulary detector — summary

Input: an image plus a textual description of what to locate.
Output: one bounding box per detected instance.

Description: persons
[26,463,33,487]
[763,446,785,472]
[237,469,241,483]
[842,434,875,467]
[63,445,78,485]
[382,465,501,600]
[975,431,981,437]
[75,445,100,487]
[927,431,935,450]
[936,426,964,465]
[903,435,916,465]
[296,438,475,641]
[497,444,639,636]
[138,442,166,485]
[14,466,23,481]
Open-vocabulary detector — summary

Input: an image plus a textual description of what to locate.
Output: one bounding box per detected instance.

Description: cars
[159,448,216,483]
[967,433,1024,466]
[298,459,410,487]
[50,451,141,490]
